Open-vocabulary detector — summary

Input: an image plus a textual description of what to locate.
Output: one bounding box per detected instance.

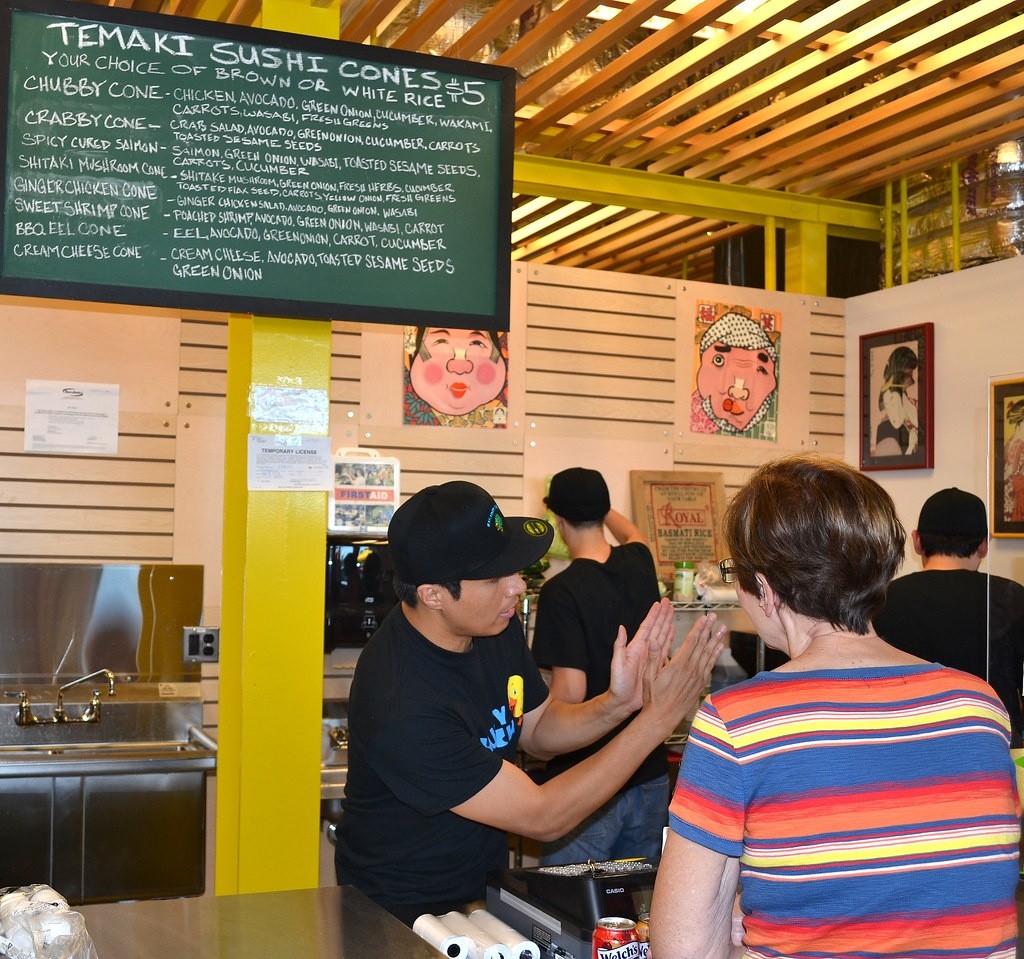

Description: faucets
[52,666,117,719]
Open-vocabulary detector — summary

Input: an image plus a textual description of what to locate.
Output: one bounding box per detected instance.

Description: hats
[388,480,555,586]
[543,468,610,520]
[917,486,988,536]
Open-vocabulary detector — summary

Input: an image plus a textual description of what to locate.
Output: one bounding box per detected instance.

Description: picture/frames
[631,469,736,595]
[857,322,936,472]
[988,372,1023,541]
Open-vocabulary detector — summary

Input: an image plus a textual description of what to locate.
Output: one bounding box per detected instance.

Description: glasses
[718,558,740,583]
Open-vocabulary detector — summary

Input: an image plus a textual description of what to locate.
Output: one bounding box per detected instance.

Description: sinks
[0,697,210,770]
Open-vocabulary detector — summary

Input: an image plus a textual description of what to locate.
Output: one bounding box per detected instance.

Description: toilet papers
[412,912,476,959]
[469,908,542,959]
[441,910,513,959]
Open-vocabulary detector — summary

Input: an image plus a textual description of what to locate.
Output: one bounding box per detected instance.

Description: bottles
[673,561,694,602]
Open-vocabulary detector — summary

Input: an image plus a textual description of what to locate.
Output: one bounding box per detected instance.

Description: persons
[870,489,1023,743]
[524,468,674,895]
[329,483,729,914]
[652,455,1023,959]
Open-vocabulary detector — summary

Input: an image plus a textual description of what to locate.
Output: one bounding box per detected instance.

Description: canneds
[673,559,695,601]
[590,915,649,959]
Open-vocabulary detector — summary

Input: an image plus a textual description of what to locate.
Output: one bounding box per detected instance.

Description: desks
[0,885,450,959]
